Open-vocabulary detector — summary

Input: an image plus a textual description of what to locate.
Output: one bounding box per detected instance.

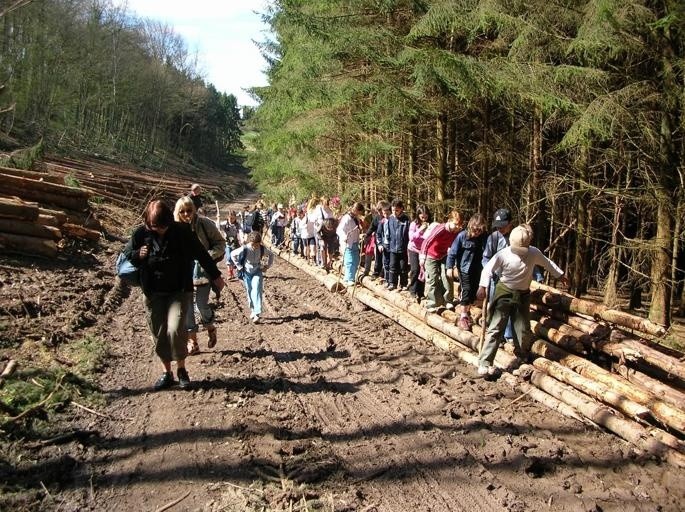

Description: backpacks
[237,245,264,279]
[246,211,259,225]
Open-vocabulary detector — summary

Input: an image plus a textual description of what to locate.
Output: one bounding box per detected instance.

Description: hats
[492,208,512,229]
[508,225,530,256]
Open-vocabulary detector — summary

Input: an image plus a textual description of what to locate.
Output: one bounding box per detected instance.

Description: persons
[476,225,569,375]
[197,206,208,218]
[336,200,543,350]
[124,200,225,390]
[187,183,204,213]
[222,198,343,280]
[230,231,274,324]
[173,196,228,355]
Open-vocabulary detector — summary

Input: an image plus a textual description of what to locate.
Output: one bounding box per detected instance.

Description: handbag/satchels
[115,251,140,286]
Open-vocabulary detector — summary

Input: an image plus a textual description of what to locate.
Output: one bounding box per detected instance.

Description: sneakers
[208,325,217,348]
[250,311,254,318]
[517,357,527,364]
[177,367,191,390]
[187,341,200,356]
[347,271,474,331]
[155,372,174,390]
[253,315,260,323]
[477,365,489,375]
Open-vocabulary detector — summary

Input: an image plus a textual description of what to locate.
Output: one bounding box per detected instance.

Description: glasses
[178,208,193,214]
